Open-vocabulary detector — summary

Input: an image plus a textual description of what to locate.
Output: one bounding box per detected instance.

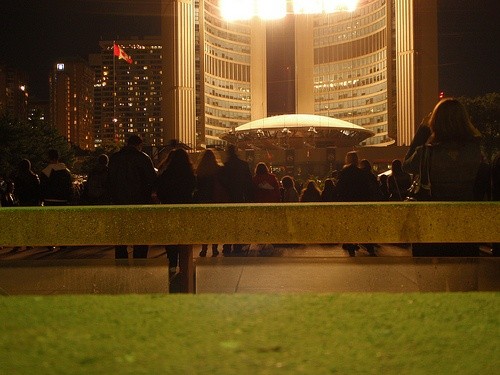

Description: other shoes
[199,248,207,256]
[349,249,355,256]
[233,244,242,249]
[212,247,219,256]
[368,248,374,255]
[222,244,231,254]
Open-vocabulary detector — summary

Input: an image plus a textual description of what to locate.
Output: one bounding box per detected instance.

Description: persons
[400,98,500,258]
[191,148,223,257]
[282,175,298,202]
[105,135,166,258]
[320,178,335,201]
[331,169,343,187]
[336,152,381,258]
[156,151,196,258]
[378,174,389,201]
[298,180,322,202]
[0,149,109,251]
[387,160,411,201]
[359,159,371,171]
[251,162,280,203]
[212,145,254,255]
